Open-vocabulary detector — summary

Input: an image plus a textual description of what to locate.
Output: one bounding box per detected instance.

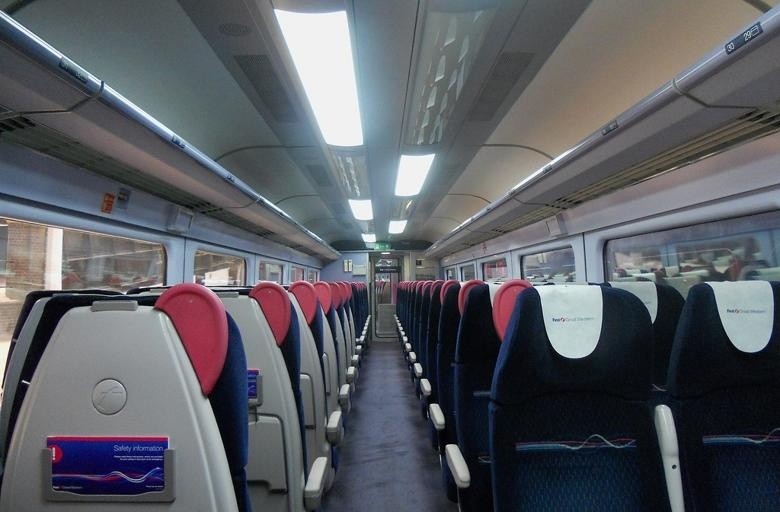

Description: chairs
[0,281,372,512]
[487,246,780,280]
[393,278,780,512]
